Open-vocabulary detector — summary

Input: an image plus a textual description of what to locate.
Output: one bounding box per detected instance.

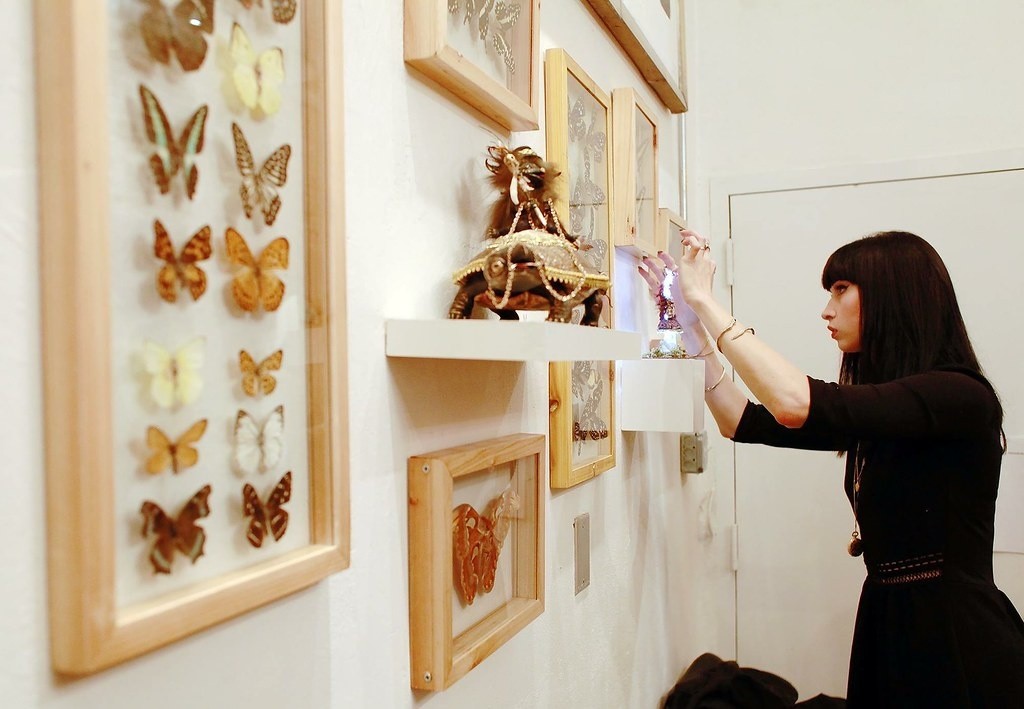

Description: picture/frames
[30,2,689,692]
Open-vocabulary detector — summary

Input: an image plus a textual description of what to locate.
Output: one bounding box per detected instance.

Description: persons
[637,228,1024,709]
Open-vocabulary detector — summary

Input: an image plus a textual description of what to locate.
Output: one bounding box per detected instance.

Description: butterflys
[452,483,522,606]
[571,359,609,447]
[136,1,300,577]
[446,0,522,76]
[567,93,608,273]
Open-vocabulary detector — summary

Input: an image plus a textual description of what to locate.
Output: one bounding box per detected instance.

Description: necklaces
[847,455,866,556]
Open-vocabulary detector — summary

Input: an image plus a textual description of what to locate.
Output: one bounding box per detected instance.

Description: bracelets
[685,334,715,358]
[715,317,755,353]
[701,365,726,392]
